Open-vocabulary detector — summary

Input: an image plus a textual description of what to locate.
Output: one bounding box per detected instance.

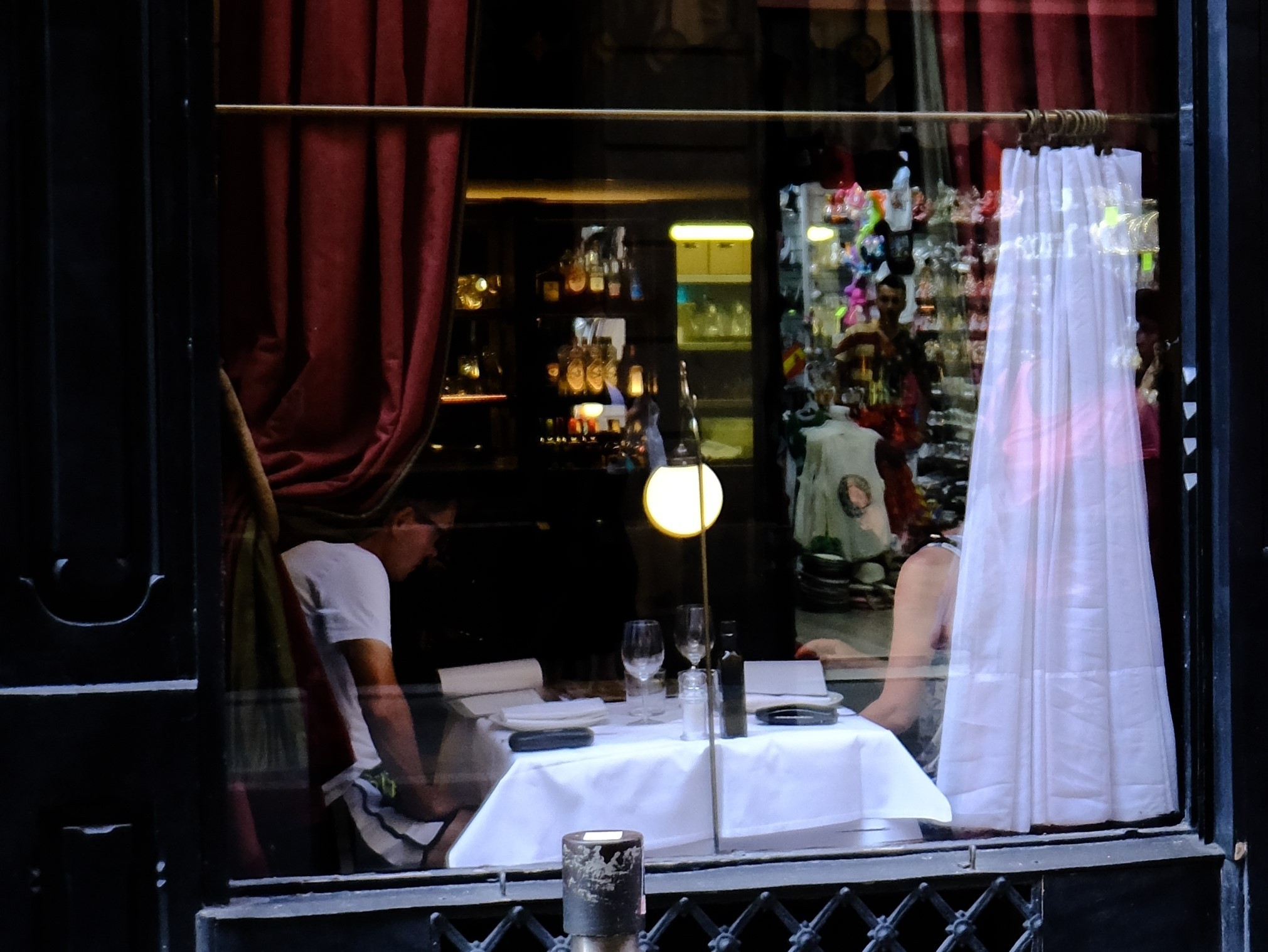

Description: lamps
[642,360,723,853]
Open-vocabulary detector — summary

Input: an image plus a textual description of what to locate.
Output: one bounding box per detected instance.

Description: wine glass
[675,606,715,710]
[621,620,665,727]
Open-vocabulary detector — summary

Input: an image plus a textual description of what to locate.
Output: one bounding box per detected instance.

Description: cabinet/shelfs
[675,239,751,282]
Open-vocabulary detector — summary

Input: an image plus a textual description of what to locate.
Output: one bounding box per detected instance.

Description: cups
[625,669,666,717]
[562,830,647,936]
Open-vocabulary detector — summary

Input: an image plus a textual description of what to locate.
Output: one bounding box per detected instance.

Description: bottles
[534,239,646,304]
[678,669,708,740]
[453,274,502,311]
[541,417,648,471]
[677,301,752,339]
[547,337,659,398]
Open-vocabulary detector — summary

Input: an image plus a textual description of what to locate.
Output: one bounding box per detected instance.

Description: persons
[872,276,930,442]
[796,429,969,790]
[270,445,473,876]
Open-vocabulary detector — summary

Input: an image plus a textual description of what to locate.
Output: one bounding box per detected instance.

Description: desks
[431,673,934,877]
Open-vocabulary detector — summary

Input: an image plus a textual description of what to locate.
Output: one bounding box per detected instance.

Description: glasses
[411,505,451,541]
